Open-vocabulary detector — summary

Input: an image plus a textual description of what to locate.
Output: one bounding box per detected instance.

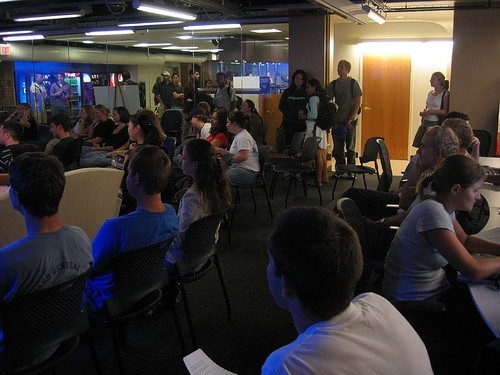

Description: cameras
[112,160,124,169]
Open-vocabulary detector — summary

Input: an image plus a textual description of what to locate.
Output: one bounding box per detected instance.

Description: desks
[467,157,500,340]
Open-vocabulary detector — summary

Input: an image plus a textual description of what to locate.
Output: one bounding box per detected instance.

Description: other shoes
[281,173,290,180]
[320,180,329,182]
[307,181,322,187]
[348,172,357,179]
[335,170,344,177]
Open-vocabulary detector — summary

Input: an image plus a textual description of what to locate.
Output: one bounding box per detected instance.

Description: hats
[161,71,170,78]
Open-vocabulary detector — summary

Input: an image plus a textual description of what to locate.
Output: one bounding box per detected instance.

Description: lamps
[361,0,387,25]
[13,9,85,21]
[116,15,185,27]
[132,0,197,20]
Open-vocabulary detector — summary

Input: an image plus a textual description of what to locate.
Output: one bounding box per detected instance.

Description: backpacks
[308,94,336,131]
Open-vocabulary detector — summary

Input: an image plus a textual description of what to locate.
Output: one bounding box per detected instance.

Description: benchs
[0,167,125,249]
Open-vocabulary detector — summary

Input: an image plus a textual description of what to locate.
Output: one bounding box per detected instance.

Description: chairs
[0,110,491,375]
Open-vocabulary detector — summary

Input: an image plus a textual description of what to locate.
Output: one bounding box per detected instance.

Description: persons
[322,60,363,180]
[0,149,95,375]
[0,61,339,242]
[412,72,450,156]
[79,143,179,327]
[164,137,234,303]
[245,109,500,374]
